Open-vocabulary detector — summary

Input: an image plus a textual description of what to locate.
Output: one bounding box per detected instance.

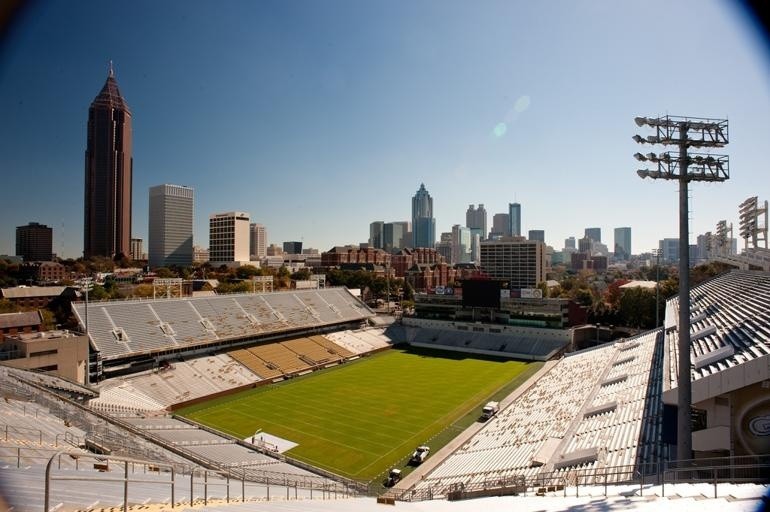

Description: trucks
[482,401,499,420]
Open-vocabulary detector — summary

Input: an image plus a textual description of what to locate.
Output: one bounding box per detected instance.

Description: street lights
[79,271,94,389]
[633,114,729,479]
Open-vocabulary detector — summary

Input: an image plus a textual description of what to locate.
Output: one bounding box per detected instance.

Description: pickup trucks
[412,447,430,463]
